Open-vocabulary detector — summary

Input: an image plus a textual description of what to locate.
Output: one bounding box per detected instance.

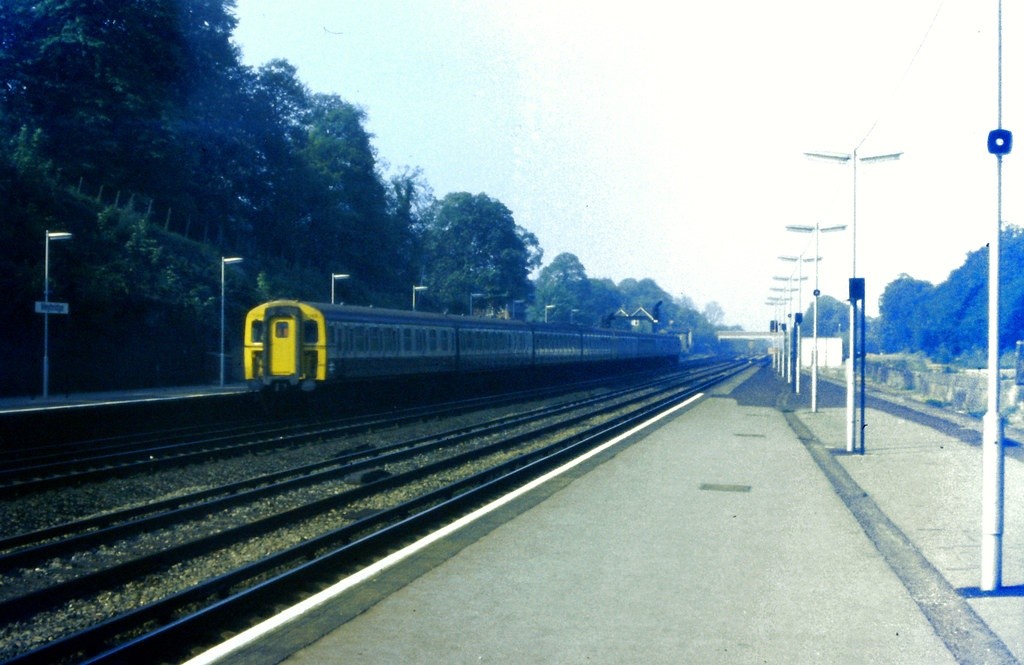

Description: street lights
[544,304,556,323]
[42,229,73,400]
[570,308,580,325]
[778,255,822,395]
[804,150,903,454]
[763,275,808,384]
[219,256,245,386]
[412,284,428,311]
[785,224,847,413]
[469,292,485,316]
[331,272,350,305]
[512,299,525,320]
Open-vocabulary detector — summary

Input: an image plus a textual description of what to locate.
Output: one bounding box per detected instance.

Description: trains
[242,296,683,423]
